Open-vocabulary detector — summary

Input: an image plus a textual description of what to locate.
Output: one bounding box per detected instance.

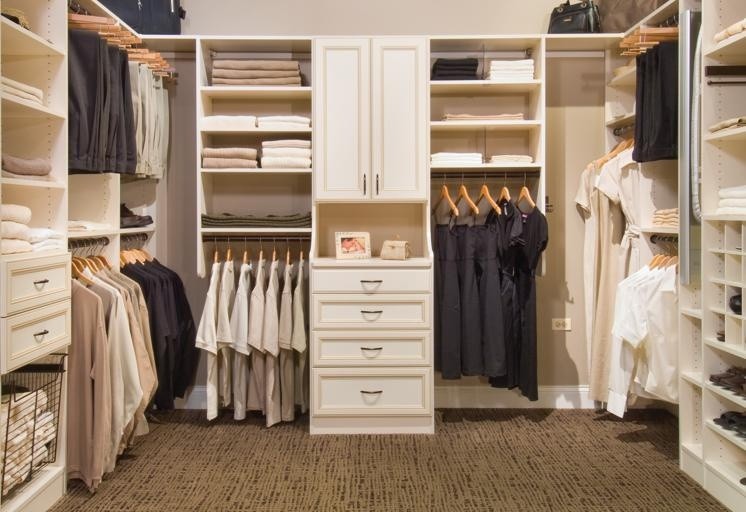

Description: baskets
[1,350,67,499]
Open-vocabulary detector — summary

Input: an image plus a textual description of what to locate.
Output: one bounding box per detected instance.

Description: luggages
[100,1,186,34]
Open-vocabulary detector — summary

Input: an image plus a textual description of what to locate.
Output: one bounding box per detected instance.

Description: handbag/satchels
[548,1,602,34]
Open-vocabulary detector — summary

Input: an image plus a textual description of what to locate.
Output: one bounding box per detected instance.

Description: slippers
[712,410,746,435]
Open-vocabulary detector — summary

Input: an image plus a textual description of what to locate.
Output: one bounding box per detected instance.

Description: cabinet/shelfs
[140,33,616,436]
[1,1,140,511]
[616,1,745,512]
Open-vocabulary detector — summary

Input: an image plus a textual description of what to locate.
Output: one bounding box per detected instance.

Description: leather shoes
[123,200,153,225]
[709,364,746,395]
[121,205,143,227]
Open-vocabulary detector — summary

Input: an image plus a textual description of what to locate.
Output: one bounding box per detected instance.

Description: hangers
[71,238,109,282]
[210,234,308,267]
[120,234,153,268]
[433,172,536,220]
[646,236,678,274]
[602,126,637,165]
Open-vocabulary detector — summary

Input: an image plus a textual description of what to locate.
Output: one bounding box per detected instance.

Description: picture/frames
[333,230,371,261]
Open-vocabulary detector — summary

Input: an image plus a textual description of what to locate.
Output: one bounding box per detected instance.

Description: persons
[341,238,355,254]
[352,238,365,253]
